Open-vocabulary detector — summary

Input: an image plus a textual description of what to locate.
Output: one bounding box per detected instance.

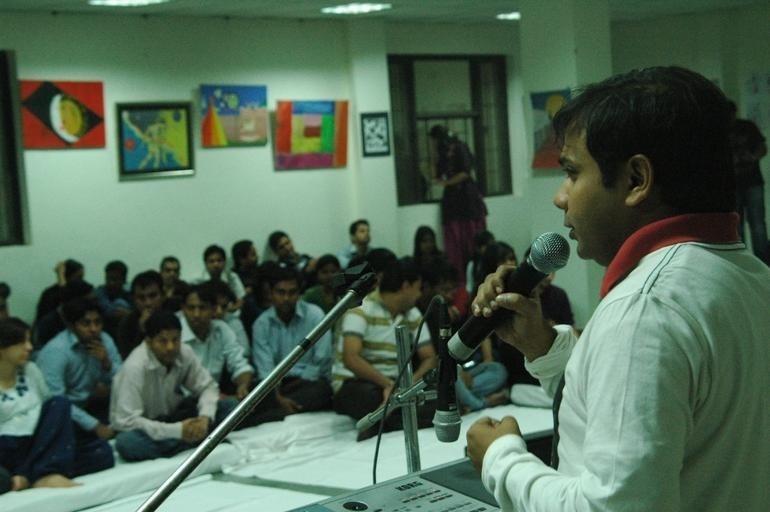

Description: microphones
[433,304,463,444]
[445,231,571,360]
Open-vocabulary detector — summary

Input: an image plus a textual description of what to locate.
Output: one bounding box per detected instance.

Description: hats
[426,126,444,138]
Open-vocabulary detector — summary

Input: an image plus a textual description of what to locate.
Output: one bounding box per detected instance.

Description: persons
[0,316,76,495]
[468,241,518,388]
[462,231,495,308]
[231,240,282,338]
[268,230,312,276]
[414,226,441,260]
[248,267,333,429]
[331,259,447,440]
[728,101,770,267]
[417,259,510,416]
[303,255,349,316]
[195,245,245,319]
[466,67,770,511]
[110,311,240,462]
[340,219,372,278]
[523,244,578,339]
[35,296,122,479]
[158,256,193,305]
[32,258,93,350]
[427,124,488,285]
[0,281,9,323]
[97,260,135,331]
[117,271,179,360]
[208,280,251,396]
[178,284,254,401]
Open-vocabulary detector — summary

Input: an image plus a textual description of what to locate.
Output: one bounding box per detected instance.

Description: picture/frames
[360,113,391,156]
[115,101,197,177]
